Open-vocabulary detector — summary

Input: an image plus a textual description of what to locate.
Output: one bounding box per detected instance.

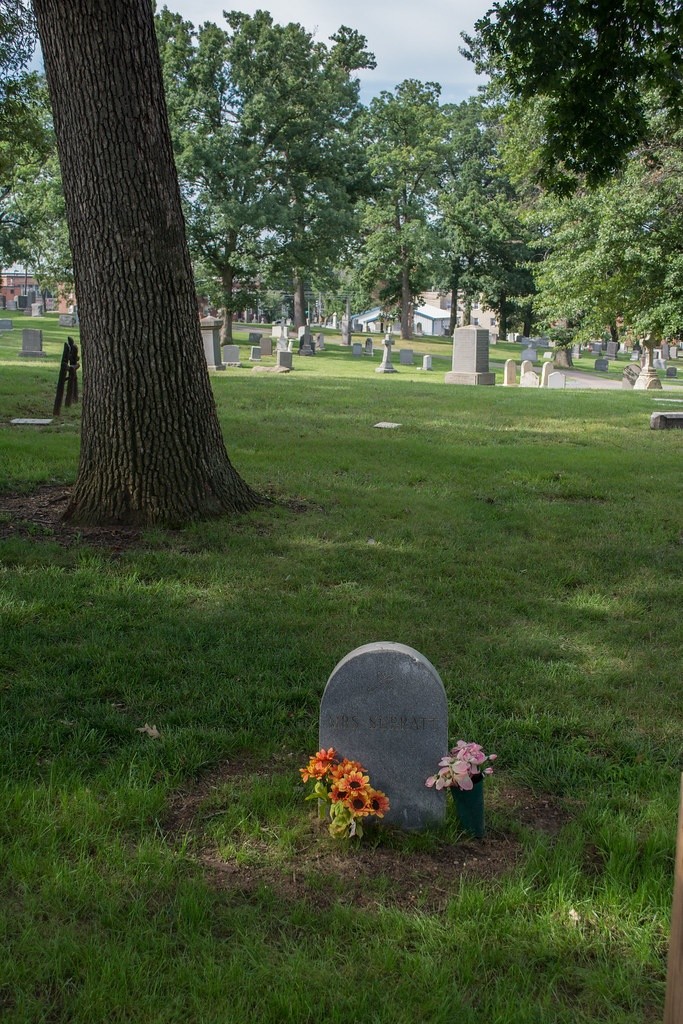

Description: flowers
[425,740,498,792]
[298,748,391,840]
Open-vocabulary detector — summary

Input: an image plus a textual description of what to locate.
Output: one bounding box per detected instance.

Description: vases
[449,780,485,838]
